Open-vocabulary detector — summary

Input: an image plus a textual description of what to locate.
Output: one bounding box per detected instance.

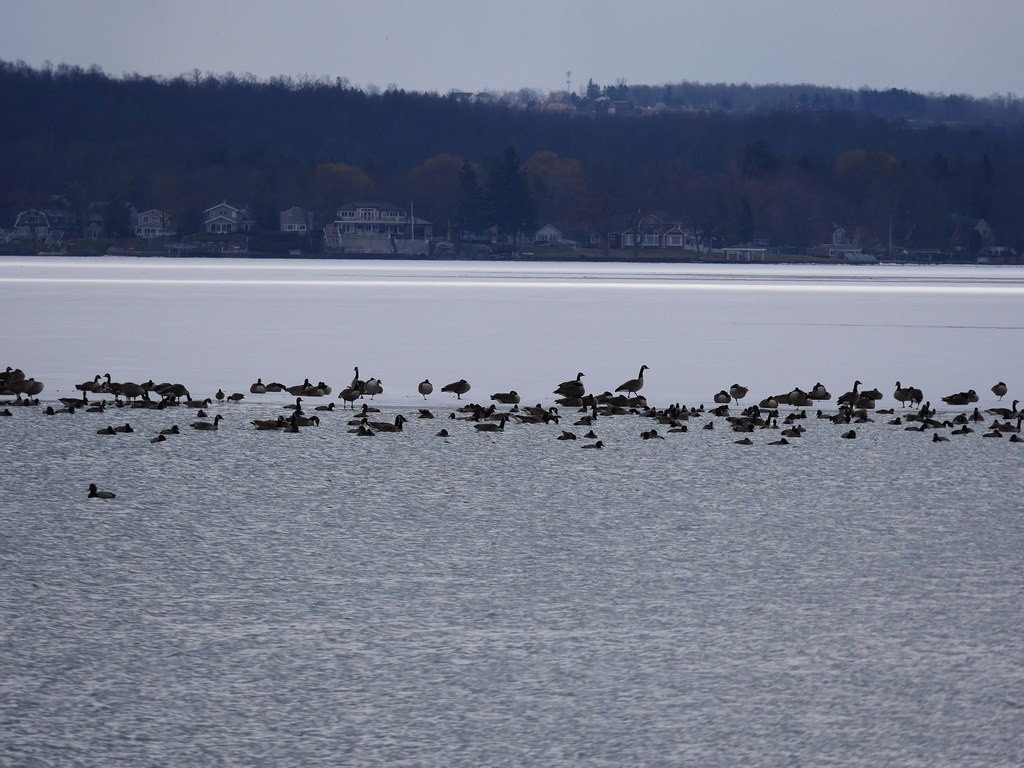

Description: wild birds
[86,484,117,498]
[0,364,1023,451]
